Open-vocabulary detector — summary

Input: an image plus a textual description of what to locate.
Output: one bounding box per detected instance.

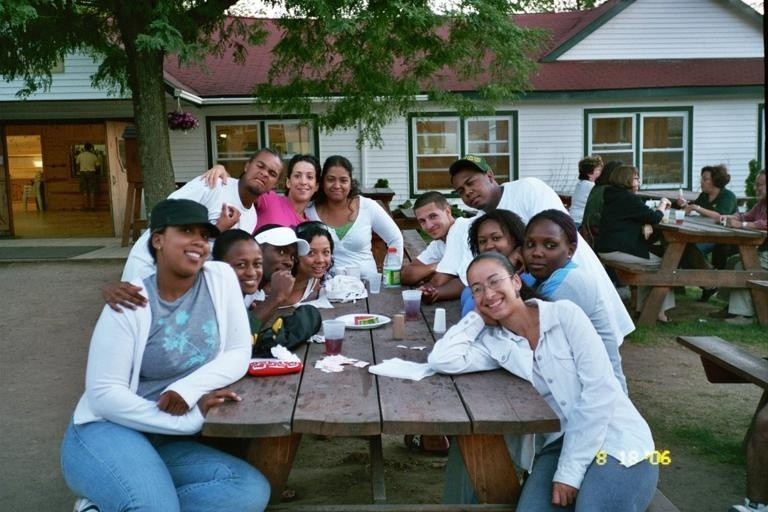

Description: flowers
[164,106,201,136]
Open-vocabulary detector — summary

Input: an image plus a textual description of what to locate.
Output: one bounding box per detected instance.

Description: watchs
[741,221,748,229]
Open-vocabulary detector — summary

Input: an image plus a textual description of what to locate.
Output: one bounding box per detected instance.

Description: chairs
[21,170,45,214]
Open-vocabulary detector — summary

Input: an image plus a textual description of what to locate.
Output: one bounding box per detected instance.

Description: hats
[150,199,221,238]
[451,154,490,172]
[254,226,309,256]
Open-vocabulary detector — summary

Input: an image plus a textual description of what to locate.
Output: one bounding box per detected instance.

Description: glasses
[472,275,513,298]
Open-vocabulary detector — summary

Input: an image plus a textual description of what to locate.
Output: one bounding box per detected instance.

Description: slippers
[405,433,450,456]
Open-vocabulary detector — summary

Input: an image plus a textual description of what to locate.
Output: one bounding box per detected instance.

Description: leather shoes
[707,305,752,319]
[634,311,681,326]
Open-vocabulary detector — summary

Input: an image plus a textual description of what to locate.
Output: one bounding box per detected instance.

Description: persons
[427,251,660,512]
[75,142,103,213]
[60,197,273,510]
[709,169,768,326]
[595,164,677,323]
[729,401,767,512]
[675,163,739,304]
[100,148,403,510]
[401,154,636,399]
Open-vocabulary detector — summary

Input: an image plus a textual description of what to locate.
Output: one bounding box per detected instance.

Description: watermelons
[354,316,379,325]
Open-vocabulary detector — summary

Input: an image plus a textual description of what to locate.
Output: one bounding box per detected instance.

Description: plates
[335,314,391,330]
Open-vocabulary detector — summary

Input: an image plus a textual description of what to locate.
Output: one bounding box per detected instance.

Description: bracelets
[695,205,700,211]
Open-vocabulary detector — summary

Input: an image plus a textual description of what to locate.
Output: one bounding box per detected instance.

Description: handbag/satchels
[252,305,320,358]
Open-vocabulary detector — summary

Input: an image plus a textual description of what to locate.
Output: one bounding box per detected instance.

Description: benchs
[400,206,421,229]
[642,485,678,512]
[673,325,766,447]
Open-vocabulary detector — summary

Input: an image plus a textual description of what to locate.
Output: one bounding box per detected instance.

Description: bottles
[391,311,406,342]
[332,265,361,279]
[433,307,446,333]
[382,248,403,290]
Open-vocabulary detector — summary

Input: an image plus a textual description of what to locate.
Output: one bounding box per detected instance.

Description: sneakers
[700,286,718,302]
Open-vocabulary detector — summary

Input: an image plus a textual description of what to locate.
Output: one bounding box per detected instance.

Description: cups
[674,210,685,226]
[661,210,669,224]
[322,319,346,358]
[368,273,382,295]
[400,290,423,318]
